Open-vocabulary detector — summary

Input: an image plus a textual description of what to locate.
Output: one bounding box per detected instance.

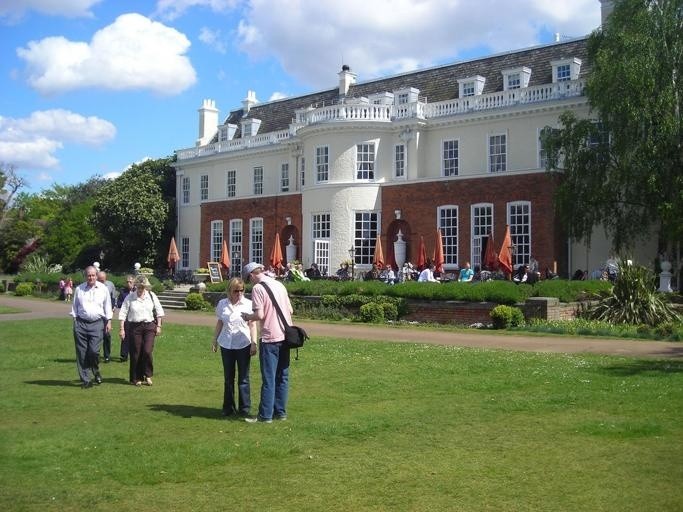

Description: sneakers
[222,410,286,423]
[136,378,152,386]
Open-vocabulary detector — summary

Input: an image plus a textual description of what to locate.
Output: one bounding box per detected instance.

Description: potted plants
[194,267,210,282]
[139,267,154,279]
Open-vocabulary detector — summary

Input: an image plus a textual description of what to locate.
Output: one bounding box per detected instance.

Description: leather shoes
[105,358,110,361]
[120,356,127,360]
[81,375,102,389]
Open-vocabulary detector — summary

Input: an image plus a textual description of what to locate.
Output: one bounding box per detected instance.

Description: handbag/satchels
[285,326,309,348]
[152,307,157,324]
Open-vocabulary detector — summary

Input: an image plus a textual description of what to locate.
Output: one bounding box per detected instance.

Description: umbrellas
[267,232,286,279]
[416,234,428,272]
[373,233,385,278]
[498,222,513,280]
[219,237,231,279]
[167,236,182,281]
[431,227,445,277]
[483,232,497,272]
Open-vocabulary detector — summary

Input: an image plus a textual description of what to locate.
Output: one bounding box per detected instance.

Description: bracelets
[245,313,250,322]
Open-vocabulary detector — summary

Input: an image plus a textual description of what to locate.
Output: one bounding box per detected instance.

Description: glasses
[235,290,244,292]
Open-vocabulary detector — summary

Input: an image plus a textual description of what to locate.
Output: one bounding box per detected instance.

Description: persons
[209,275,258,424]
[239,261,295,424]
[58,265,165,389]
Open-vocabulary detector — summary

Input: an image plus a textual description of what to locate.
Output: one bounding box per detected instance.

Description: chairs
[410,272,419,282]
[355,271,368,281]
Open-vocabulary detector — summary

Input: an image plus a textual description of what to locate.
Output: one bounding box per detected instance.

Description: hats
[242,263,264,283]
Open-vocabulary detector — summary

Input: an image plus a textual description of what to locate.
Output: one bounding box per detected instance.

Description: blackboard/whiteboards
[207,262,223,284]
